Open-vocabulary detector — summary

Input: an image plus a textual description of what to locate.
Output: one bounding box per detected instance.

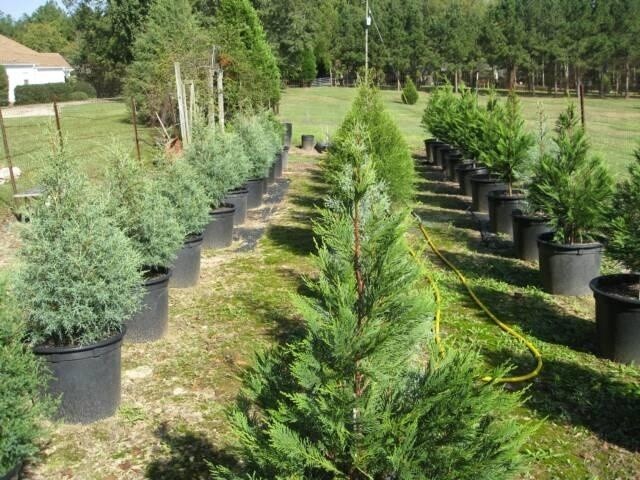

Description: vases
[302,134,314,152]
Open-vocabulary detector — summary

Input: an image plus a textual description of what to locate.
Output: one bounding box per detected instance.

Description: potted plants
[0,107,293,480]
[418,82,640,371]
